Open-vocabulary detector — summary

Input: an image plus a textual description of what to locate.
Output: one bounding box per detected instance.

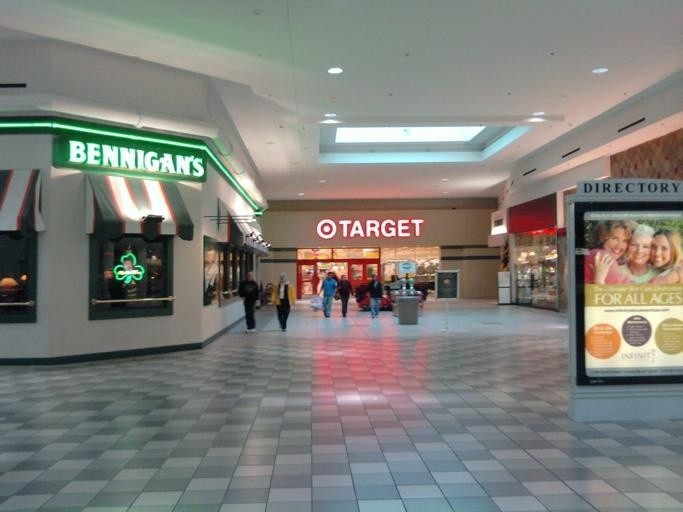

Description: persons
[587,224,665,286]
[336,274,354,317]
[640,227,682,286]
[203,245,219,306]
[237,270,259,333]
[318,272,337,317]
[363,274,383,319]
[583,216,637,284]
[272,271,296,331]
[404,273,410,290]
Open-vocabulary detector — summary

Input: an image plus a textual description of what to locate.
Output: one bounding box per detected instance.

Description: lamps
[245,231,271,250]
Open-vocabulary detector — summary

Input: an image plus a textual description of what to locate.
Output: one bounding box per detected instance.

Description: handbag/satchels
[310,294,323,311]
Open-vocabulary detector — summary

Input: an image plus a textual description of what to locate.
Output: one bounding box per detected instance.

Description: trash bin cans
[396,295,421,324]
